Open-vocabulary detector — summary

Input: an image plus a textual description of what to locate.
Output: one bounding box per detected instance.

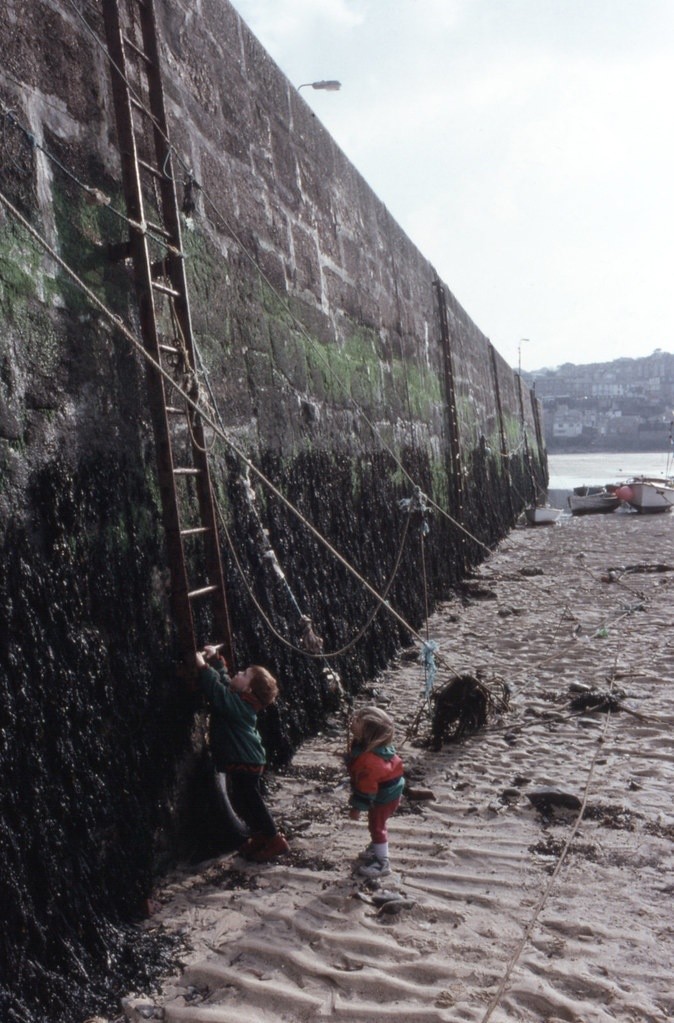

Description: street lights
[518,337,530,376]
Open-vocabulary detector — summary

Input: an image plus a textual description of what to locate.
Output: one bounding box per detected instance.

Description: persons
[343,706,405,878]
[195,644,291,862]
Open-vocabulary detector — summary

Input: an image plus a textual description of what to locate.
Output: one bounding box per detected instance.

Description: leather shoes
[240,831,290,862]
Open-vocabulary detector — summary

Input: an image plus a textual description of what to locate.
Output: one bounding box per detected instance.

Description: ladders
[103,0,238,710]
[433,281,468,559]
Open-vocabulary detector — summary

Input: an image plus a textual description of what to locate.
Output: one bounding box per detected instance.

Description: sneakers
[358,841,376,860]
[359,857,392,876]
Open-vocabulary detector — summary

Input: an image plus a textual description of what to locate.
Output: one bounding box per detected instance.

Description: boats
[618,472,674,515]
[567,482,623,516]
